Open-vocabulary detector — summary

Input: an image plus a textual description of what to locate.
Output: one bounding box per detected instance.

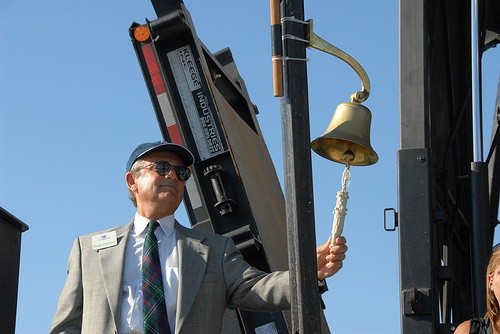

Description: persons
[48,140,347,334]
[453,240,500,334]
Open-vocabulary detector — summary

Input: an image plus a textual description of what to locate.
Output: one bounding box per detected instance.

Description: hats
[126,141,196,169]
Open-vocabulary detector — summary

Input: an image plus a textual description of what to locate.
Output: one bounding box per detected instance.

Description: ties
[142,220,172,334]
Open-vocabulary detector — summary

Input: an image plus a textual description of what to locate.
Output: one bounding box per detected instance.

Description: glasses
[133,161,191,182]
